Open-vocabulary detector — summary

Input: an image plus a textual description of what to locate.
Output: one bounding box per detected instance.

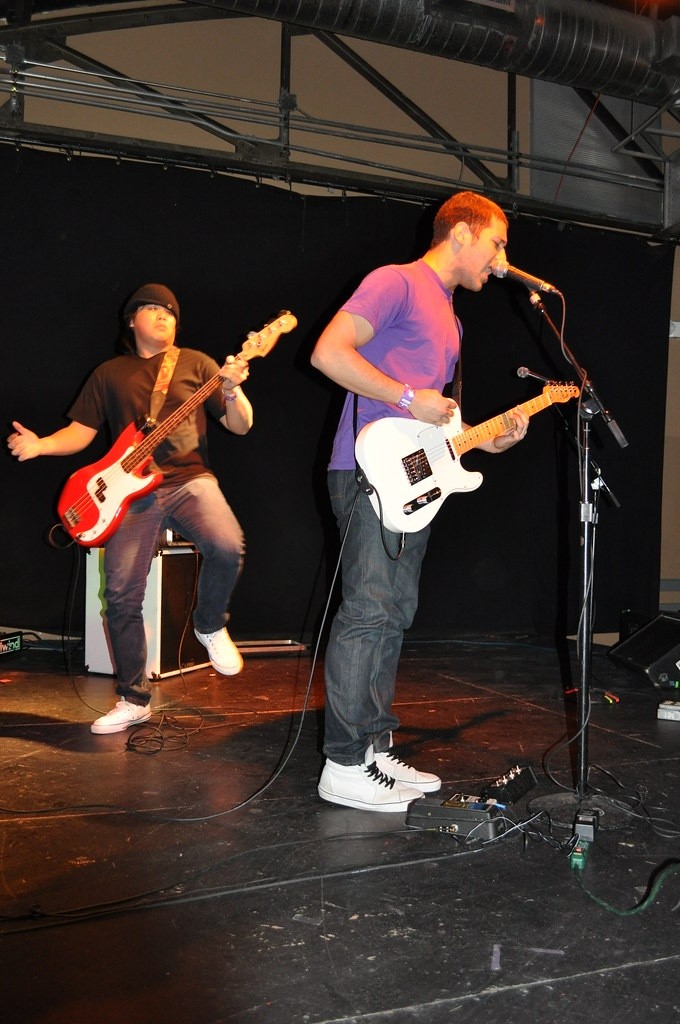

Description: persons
[8,282,253,734]
[310,191,530,813]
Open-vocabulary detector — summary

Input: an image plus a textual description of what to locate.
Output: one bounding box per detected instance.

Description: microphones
[517,367,550,382]
[492,260,558,293]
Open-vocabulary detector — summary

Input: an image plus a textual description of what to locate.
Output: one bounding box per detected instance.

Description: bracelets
[396,383,414,409]
[225,393,238,401]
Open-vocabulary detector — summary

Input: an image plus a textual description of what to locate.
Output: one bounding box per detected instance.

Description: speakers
[607,614,680,690]
[84,548,214,678]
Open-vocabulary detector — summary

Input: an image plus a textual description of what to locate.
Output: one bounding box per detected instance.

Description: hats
[121,282,180,329]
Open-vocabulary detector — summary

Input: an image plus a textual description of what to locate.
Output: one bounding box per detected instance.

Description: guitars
[57,310,299,548]
[354,380,580,535]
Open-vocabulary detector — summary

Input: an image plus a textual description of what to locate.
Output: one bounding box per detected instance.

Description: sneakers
[374,752,442,795]
[193,625,244,676]
[90,700,152,735]
[317,743,427,813]
